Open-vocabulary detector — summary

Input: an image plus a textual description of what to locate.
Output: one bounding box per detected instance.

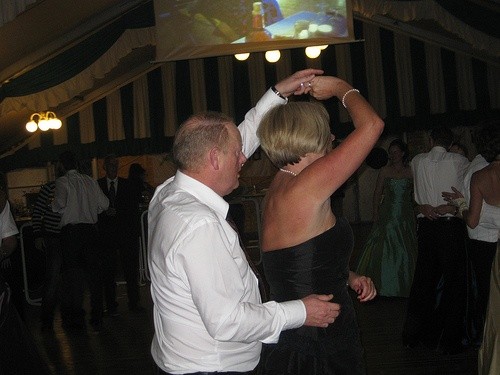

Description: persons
[372,124,500,375]
[260,76,386,375]
[147,69,342,375]
[0,150,157,375]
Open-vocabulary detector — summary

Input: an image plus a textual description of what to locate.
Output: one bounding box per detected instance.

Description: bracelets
[271,84,286,100]
[342,89,360,109]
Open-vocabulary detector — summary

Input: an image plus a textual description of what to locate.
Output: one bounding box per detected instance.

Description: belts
[420,217,463,222]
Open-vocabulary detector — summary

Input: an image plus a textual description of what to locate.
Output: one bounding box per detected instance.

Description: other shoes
[129,304,146,312]
[105,308,119,315]
[88,318,103,326]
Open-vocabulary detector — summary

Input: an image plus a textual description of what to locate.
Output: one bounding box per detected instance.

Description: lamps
[25,110,62,133]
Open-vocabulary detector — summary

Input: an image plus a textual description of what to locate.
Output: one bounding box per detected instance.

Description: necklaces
[280,167,297,176]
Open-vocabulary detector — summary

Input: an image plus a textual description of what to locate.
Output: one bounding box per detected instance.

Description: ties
[110,181,115,208]
[226,213,265,288]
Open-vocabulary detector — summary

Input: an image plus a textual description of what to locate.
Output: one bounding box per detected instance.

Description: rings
[309,81,311,87]
